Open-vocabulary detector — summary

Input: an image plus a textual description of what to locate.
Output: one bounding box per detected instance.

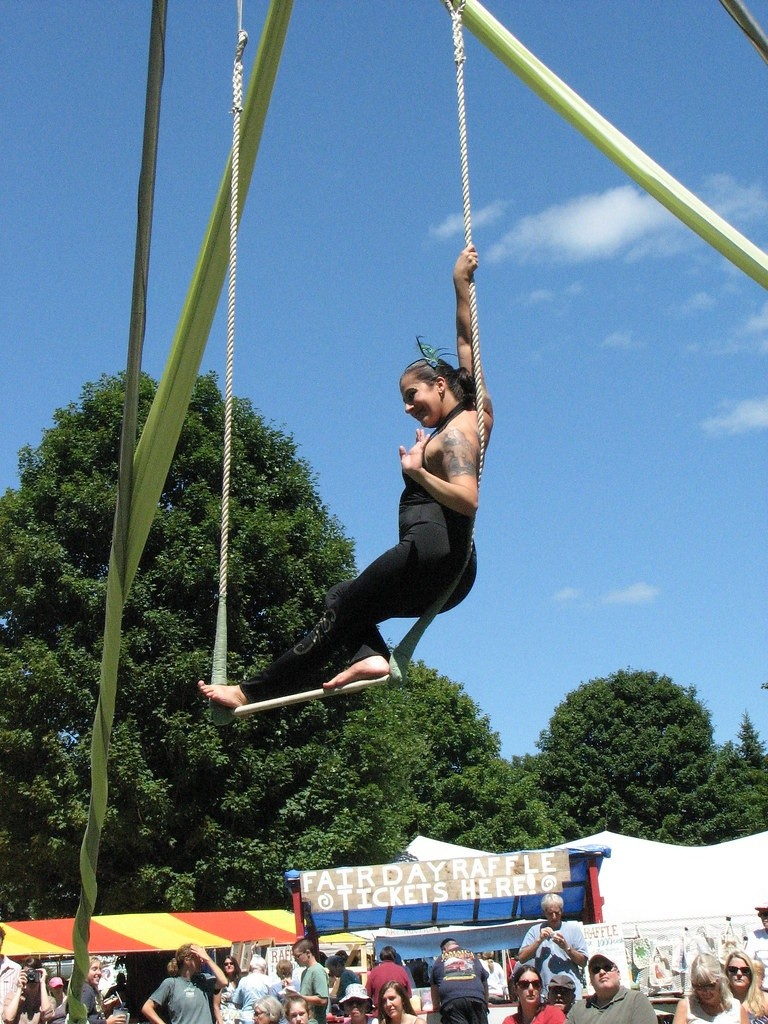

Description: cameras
[23,969,40,983]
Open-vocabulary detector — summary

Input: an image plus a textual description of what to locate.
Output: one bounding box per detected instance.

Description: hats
[548,976,576,993]
[339,983,372,1003]
[588,953,621,976]
[440,938,456,948]
[754,903,768,911]
[49,976,64,988]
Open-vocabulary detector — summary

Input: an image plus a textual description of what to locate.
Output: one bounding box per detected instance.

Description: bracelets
[565,946,571,953]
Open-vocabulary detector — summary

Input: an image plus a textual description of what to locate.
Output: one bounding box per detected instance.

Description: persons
[1,894,768,1024]
[198,244,493,708]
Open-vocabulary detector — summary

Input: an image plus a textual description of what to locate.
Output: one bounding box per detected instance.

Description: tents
[0,909,368,955]
[393,829,768,920]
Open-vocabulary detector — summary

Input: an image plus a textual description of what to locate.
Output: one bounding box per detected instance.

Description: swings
[200,0,489,735]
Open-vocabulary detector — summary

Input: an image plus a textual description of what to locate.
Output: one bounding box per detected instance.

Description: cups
[112,1006,129,1024]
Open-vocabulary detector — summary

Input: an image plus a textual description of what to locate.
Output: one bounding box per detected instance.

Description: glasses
[727,966,751,975]
[590,963,615,974]
[517,979,541,990]
[692,982,717,990]
[224,962,234,966]
[255,1012,264,1017]
[293,952,304,957]
[758,911,768,917]
